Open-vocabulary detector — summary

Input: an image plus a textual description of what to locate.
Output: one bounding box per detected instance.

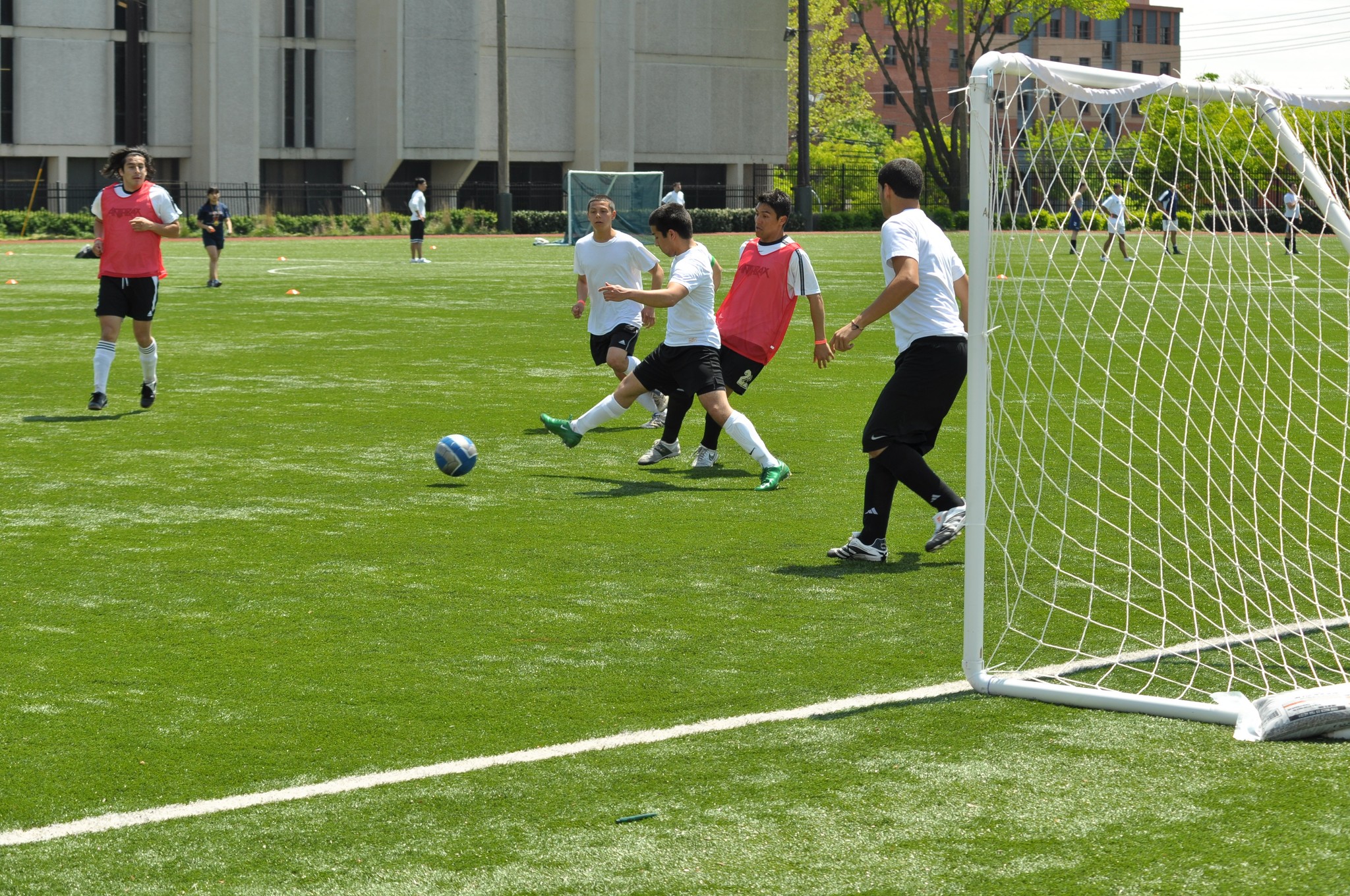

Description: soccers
[434,434,477,477]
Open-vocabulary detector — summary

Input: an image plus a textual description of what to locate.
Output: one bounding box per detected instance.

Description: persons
[1158,182,1185,255]
[638,188,834,465]
[1100,183,1137,262]
[826,159,969,562]
[572,194,669,428]
[87,146,182,411]
[196,186,232,288]
[540,203,793,490]
[408,178,431,263]
[1284,182,1303,255]
[1070,181,1087,255]
[661,183,685,208]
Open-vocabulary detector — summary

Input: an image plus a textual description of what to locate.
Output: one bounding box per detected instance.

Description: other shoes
[207,280,222,287]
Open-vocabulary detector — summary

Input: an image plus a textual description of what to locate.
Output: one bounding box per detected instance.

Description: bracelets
[94,238,103,243]
[815,338,827,345]
[851,319,864,331]
[418,216,421,218]
[577,300,585,307]
[1111,213,1113,218]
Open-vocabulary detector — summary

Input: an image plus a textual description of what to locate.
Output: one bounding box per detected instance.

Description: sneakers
[650,389,670,412]
[1294,250,1302,254]
[541,413,583,449]
[1100,255,1109,262]
[1165,249,1171,255]
[411,258,420,262]
[418,258,431,263]
[640,409,667,429]
[827,532,888,562]
[1125,257,1137,262]
[925,497,966,552]
[1070,250,1081,255]
[1285,251,1291,255]
[637,438,681,465]
[691,443,719,467]
[1173,250,1185,255]
[755,460,793,491]
[88,392,108,410]
[140,373,157,408]
